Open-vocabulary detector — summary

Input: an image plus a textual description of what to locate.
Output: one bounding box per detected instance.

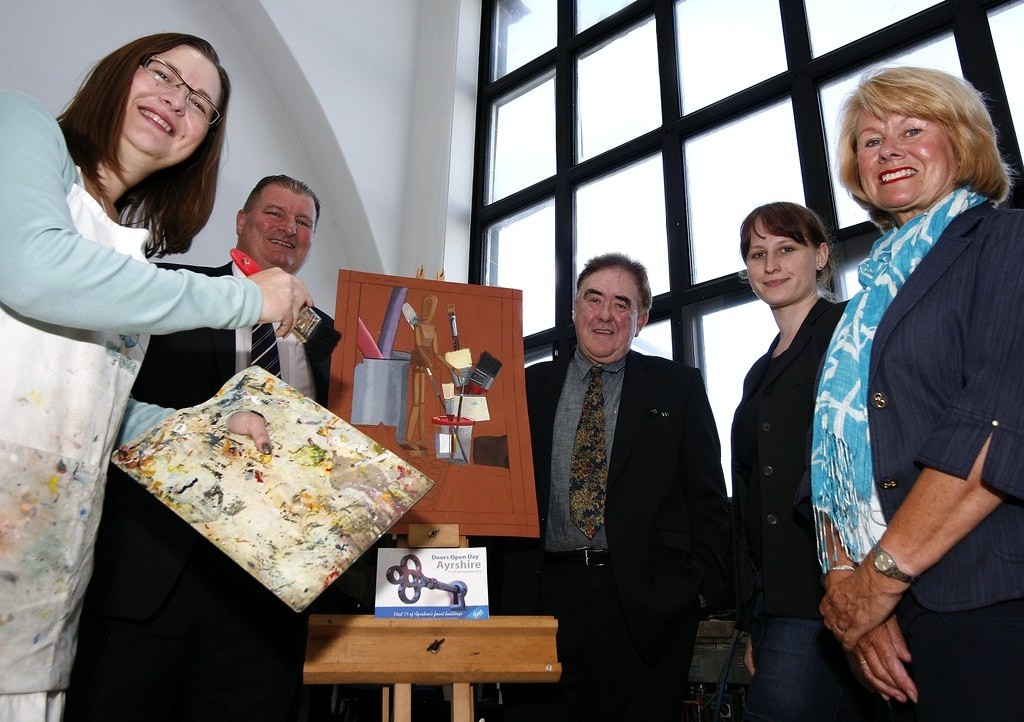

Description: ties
[250,322,282,379]
[568,365,609,541]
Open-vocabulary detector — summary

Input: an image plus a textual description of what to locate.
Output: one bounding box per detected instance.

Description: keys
[385,554,459,604]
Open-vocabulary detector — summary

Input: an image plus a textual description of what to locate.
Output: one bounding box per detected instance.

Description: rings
[860,660,867,666]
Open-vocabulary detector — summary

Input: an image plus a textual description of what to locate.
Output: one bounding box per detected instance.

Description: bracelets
[821,565,855,583]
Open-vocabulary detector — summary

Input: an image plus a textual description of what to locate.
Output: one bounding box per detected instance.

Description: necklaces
[97,194,107,214]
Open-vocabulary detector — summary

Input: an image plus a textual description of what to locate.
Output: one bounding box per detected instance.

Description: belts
[547,549,610,567]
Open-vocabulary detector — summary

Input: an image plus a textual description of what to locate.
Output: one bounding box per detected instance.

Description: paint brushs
[448,304,460,350]
[426,367,470,464]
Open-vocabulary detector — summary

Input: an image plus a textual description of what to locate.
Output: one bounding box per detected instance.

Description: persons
[731,203,849,722]
[60,174,342,722]
[795,72,1023,722]
[0,31,315,722]
[523,254,730,722]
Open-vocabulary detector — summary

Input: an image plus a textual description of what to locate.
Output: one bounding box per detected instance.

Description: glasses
[140,54,223,128]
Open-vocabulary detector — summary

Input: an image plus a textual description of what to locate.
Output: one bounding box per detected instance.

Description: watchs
[871,544,916,583]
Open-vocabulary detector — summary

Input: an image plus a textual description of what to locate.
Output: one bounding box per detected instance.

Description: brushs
[445,347,474,396]
[468,351,503,395]
[229,248,342,363]
